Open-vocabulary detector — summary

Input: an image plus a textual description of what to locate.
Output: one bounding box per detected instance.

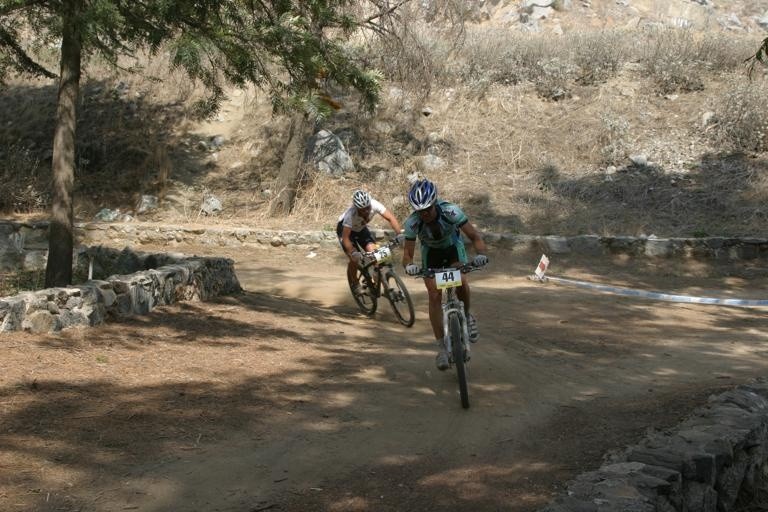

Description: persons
[400,178,489,371]
[336,190,403,296]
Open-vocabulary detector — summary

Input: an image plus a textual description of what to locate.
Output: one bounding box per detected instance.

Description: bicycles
[406,259,490,408]
[347,240,414,328]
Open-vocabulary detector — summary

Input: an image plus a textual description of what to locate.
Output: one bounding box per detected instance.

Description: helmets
[353,191,370,209]
[408,179,437,211]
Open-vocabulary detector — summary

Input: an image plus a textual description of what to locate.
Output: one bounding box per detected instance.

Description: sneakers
[466,314,480,343]
[435,352,449,370]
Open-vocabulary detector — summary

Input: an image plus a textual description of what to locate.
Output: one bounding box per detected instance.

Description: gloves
[473,255,488,266]
[396,234,405,243]
[406,263,420,275]
